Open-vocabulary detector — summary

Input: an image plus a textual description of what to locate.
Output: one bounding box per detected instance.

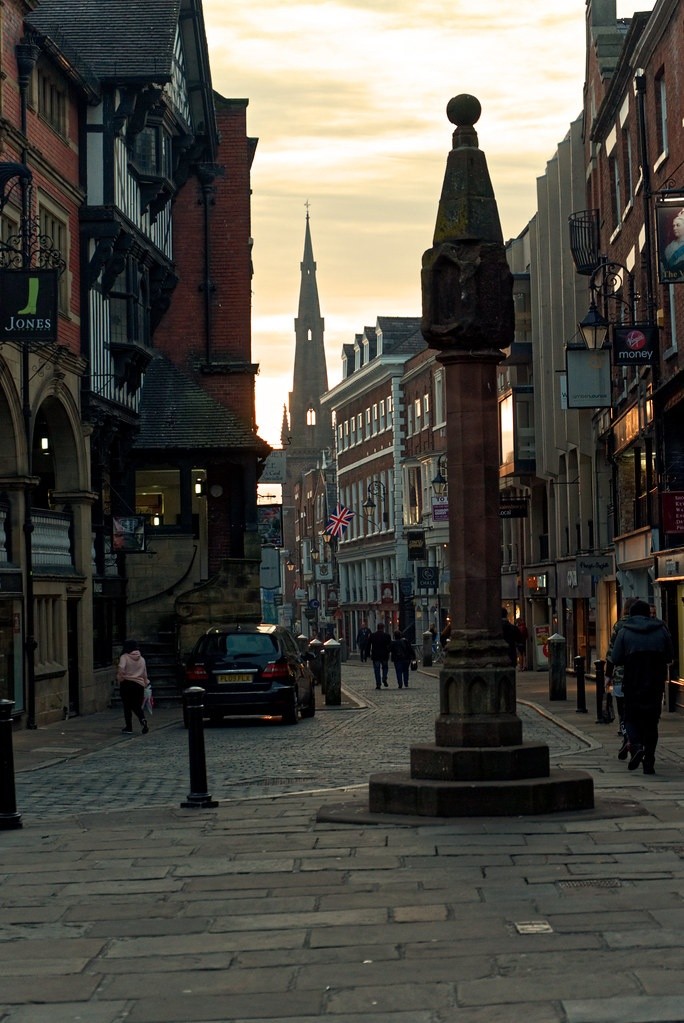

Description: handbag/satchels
[147,685,153,714]
[141,688,148,708]
[411,661,418,672]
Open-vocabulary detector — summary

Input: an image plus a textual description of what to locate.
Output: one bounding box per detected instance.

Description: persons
[391,630,411,689]
[501,607,524,656]
[366,623,391,689]
[429,622,437,642]
[116,640,150,735]
[310,630,336,641]
[356,622,372,662]
[604,597,675,774]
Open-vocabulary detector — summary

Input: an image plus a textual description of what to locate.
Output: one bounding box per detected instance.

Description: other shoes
[618,734,631,760]
[404,684,408,687]
[376,686,380,689]
[398,685,402,688]
[643,752,656,774]
[628,746,644,770]
[382,679,388,687]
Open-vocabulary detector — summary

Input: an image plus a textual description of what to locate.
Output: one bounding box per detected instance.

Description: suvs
[182,622,317,725]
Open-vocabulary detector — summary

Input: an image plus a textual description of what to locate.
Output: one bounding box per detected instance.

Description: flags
[325,503,355,538]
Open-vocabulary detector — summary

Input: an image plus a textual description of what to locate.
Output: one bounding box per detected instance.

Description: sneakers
[140,718,149,735]
[122,727,133,733]
